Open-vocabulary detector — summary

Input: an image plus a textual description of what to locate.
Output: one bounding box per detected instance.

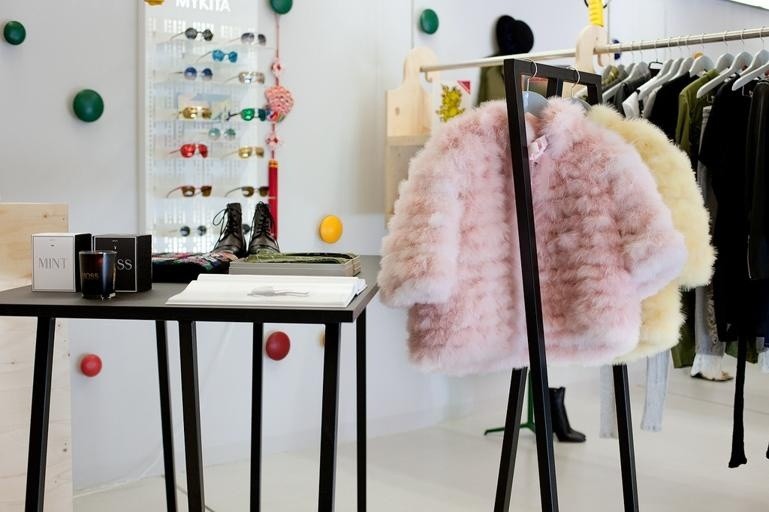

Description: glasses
[170,144,208,158]
[227,147,264,159]
[225,187,269,197]
[226,72,265,84]
[200,50,237,62]
[227,109,266,121]
[209,128,235,140]
[233,33,266,47]
[167,186,211,196]
[178,108,211,119]
[171,28,213,41]
[175,68,212,81]
[181,226,206,236]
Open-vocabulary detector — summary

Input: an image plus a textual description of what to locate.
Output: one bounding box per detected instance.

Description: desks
[0,252,381,512]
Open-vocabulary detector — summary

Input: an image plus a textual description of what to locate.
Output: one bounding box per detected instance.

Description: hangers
[521,57,551,119]
[602,25,769,103]
[567,66,592,111]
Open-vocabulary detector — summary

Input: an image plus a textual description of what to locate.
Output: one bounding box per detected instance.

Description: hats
[495,16,533,55]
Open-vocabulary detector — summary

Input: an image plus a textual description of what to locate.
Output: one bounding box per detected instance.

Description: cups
[78,251,116,303]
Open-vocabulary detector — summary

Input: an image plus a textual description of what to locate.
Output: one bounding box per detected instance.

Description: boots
[549,386,586,442]
[212,203,247,258]
[249,203,279,253]
